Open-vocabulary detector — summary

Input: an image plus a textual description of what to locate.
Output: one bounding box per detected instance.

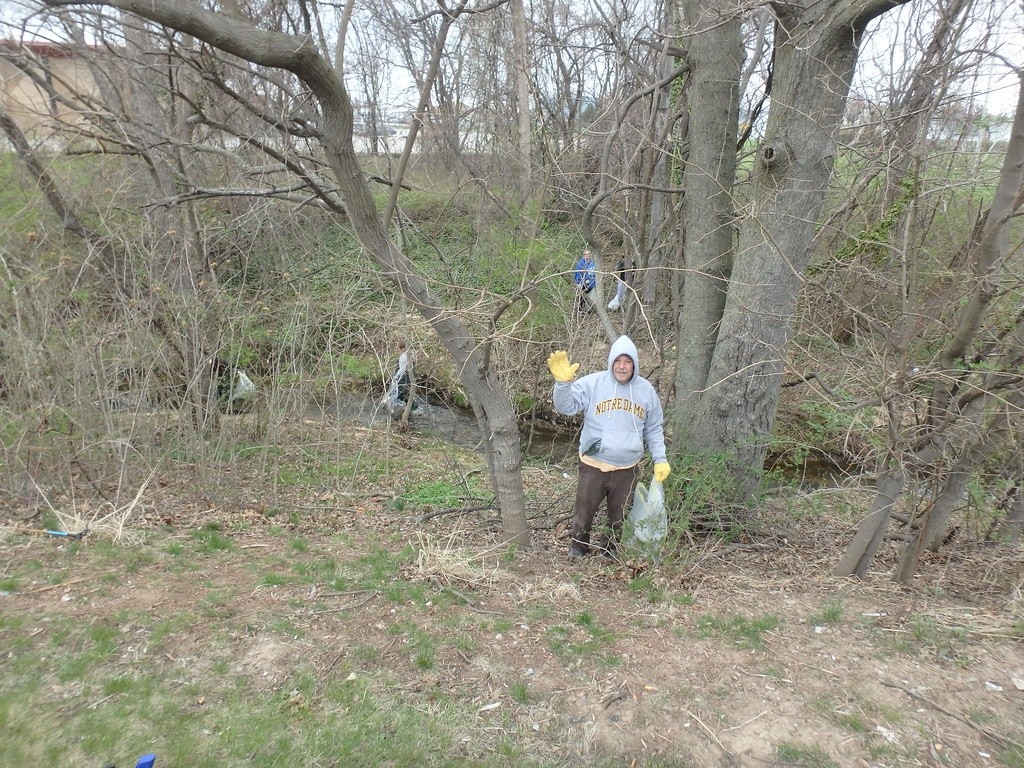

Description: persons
[571,251,596,316]
[617,252,637,306]
[390,342,423,416]
[547,335,671,565]
[209,357,244,413]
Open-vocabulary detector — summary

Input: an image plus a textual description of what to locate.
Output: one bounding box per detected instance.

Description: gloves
[653,462,671,483]
[547,350,580,383]
